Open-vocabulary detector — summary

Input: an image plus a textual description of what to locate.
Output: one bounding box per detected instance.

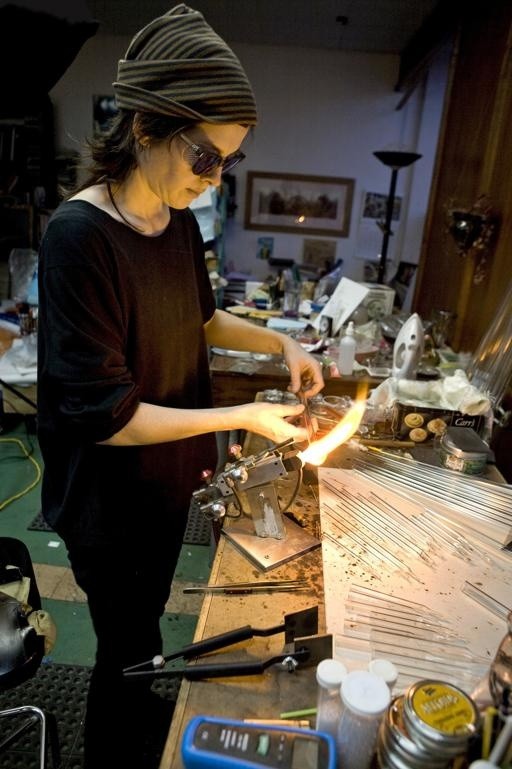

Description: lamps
[372,142,423,280]
[439,191,502,286]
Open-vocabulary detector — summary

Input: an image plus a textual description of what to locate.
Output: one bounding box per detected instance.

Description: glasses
[176,131,246,176]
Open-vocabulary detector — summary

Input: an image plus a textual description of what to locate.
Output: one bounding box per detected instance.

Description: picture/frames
[242,169,355,237]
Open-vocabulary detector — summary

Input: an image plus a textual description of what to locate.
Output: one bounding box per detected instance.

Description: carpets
[28,484,212,545]
[1,662,182,769]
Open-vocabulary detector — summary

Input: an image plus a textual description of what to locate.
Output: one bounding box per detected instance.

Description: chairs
[0,535,60,768]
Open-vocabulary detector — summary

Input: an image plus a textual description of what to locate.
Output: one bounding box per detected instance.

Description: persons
[35,4,328,769]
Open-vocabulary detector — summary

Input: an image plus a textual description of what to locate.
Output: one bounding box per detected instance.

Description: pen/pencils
[482,707,512,764]
[15,303,34,335]
[244,708,317,727]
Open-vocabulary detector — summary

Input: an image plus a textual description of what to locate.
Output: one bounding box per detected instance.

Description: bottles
[316,658,398,769]
[18,302,33,337]
[338,322,356,376]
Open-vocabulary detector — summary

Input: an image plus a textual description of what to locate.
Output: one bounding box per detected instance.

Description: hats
[111,4,259,128]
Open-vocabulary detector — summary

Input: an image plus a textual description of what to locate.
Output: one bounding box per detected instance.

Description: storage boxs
[357,283,397,322]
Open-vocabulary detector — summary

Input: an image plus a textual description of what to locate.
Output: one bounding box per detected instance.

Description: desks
[160,390,512,769]
[200,317,388,407]
